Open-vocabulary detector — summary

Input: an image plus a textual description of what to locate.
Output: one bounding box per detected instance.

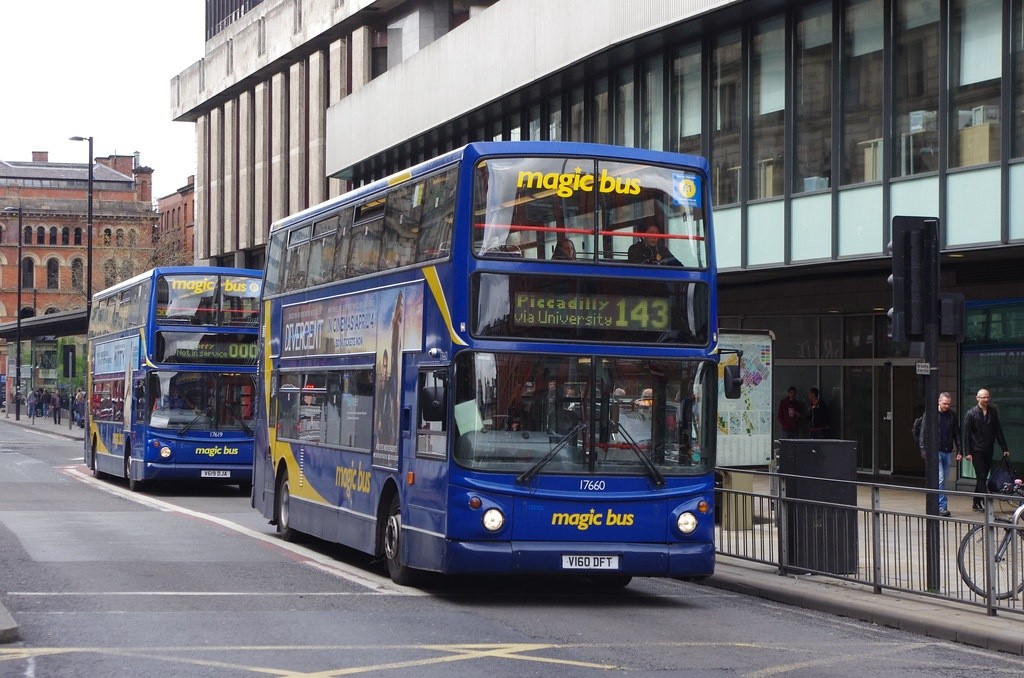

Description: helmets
[612,388,626,404]
[642,388,653,399]
[520,379,536,397]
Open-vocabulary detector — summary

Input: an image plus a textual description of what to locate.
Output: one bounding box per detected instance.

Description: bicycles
[957,481,1023,599]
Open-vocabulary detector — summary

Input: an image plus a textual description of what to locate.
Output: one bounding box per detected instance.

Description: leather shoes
[972,500,986,512]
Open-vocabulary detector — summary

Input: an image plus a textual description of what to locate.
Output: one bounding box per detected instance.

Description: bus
[83,266,264,492]
[249,141,747,591]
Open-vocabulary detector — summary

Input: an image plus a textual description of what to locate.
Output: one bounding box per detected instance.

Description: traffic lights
[886,216,894,322]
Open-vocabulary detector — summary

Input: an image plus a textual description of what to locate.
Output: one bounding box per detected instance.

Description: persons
[25,388,50,417]
[777,386,802,437]
[504,375,699,462]
[72,392,85,429]
[791,388,830,439]
[374,290,404,445]
[551,239,578,261]
[963,388,1010,511]
[920,391,963,516]
[50,389,62,425]
[626,220,685,268]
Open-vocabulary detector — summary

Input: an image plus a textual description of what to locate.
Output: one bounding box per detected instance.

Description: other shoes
[938,510,951,516]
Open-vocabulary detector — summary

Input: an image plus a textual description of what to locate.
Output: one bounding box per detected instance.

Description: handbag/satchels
[988,454,1011,492]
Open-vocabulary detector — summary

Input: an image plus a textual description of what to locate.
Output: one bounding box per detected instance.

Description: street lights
[69,135,94,327]
[5,206,22,422]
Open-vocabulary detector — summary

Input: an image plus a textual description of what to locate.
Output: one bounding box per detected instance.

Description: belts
[938,448,952,453]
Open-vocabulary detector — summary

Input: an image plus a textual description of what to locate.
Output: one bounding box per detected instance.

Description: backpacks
[911,415,924,441]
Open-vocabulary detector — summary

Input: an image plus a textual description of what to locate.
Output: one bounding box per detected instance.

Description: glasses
[977,396,991,400]
